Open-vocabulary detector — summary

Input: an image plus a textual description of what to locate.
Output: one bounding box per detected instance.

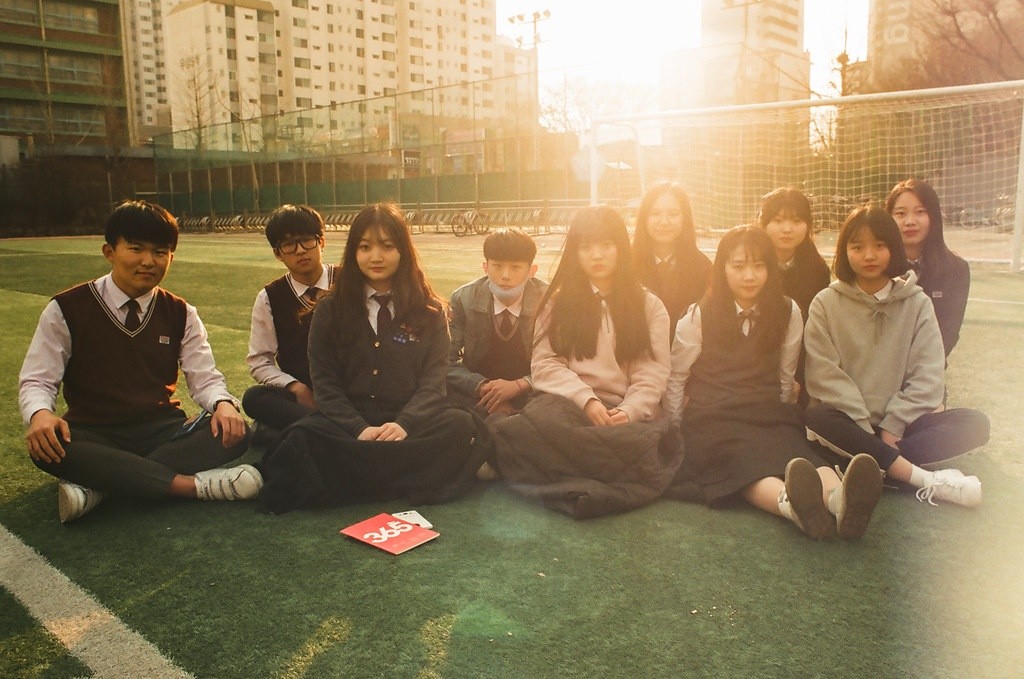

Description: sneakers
[60,481,107,523]
[194,464,269,502]
[915,468,983,509]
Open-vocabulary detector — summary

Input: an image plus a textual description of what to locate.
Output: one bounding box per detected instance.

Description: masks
[487,270,530,298]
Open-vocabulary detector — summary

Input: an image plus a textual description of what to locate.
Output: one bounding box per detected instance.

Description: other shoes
[786,458,835,538]
[836,452,883,539]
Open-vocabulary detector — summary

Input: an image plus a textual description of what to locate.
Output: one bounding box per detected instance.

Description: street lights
[508,9,552,169]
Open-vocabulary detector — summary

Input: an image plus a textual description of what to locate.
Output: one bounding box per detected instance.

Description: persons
[481,204,685,516]
[443,226,552,422]
[629,181,712,352]
[18,201,266,524]
[885,178,970,412]
[659,224,883,541]
[803,205,989,507]
[246,200,492,516]
[243,203,343,446]
[752,188,831,410]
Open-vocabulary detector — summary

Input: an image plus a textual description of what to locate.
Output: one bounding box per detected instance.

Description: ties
[307,286,318,306]
[125,301,142,331]
[500,308,513,337]
[371,294,392,341]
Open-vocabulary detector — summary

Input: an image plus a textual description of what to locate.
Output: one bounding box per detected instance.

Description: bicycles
[450,209,491,237]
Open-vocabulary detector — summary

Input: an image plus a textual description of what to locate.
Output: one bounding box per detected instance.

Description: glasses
[278,232,320,255]
